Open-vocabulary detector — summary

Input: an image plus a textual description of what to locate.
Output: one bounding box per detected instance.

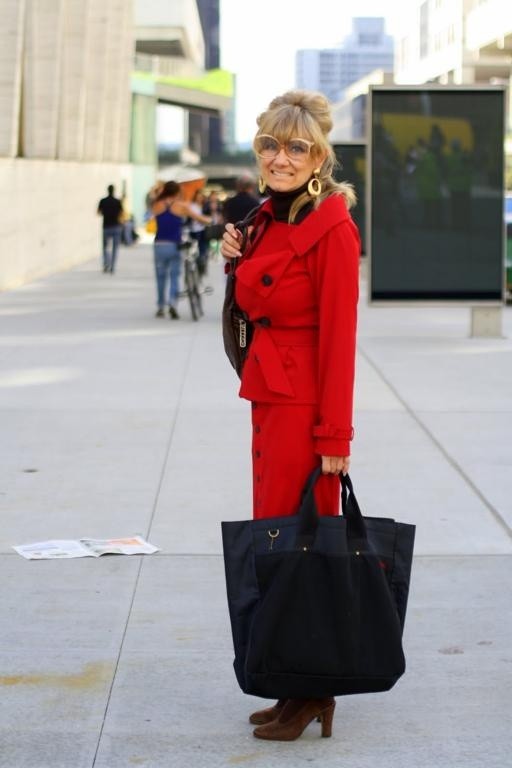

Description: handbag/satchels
[221,198,267,381]
[220,463,417,701]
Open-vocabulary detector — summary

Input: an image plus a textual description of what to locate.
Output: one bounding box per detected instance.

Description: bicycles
[176,218,214,321]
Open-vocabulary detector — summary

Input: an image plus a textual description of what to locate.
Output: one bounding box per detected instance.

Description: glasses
[252,133,316,160]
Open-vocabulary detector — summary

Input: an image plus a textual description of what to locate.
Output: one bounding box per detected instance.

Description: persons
[151,178,214,320]
[95,170,260,290]
[218,87,362,743]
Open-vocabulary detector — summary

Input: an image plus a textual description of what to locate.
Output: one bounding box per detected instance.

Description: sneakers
[155,306,180,321]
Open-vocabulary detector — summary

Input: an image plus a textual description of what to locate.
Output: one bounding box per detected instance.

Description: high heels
[248,697,336,743]
